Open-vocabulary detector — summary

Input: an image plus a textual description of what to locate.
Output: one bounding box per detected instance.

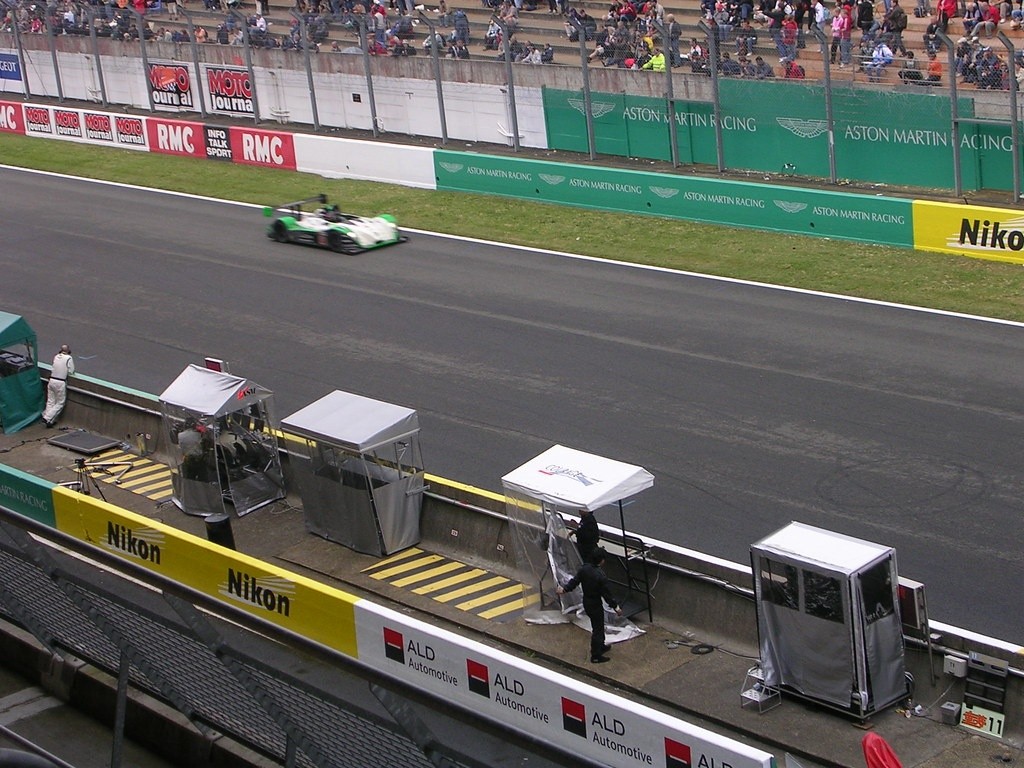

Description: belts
[49,376,65,382]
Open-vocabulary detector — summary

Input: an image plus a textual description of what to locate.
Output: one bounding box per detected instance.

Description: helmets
[324,205,341,221]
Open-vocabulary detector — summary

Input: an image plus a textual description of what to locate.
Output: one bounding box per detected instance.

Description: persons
[313,208,326,218]
[41,345,75,426]
[0,0,1024,91]
[569,510,601,559]
[178,419,208,481]
[556,547,623,662]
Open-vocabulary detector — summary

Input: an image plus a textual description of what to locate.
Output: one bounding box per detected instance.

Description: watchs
[578,525,581,528]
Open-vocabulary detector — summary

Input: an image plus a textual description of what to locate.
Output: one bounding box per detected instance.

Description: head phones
[59,344,71,355]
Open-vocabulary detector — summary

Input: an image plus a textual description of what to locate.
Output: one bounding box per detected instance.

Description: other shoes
[590,655,610,663]
[601,644,611,656]
[45,423,53,428]
[41,418,57,425]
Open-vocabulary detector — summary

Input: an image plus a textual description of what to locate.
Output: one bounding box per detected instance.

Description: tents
[157,364,286,517]
[0,310,45,435]
[501,443,656,623]
[749,519,909,714]
[280,390,427,558]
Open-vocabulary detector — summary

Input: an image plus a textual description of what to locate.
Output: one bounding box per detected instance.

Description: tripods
[75,458,107,503]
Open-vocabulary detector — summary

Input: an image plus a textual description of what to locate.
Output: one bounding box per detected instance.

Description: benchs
[0,0,1024,91]
